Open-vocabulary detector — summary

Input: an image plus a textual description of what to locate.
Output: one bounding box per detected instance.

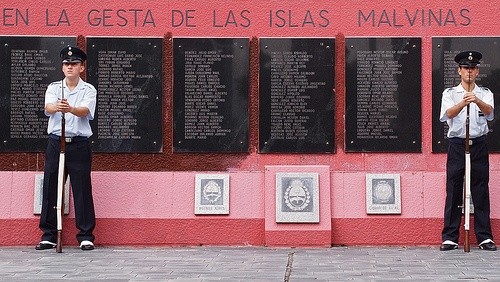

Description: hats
[59,46,88,63]
[454,51,483,67]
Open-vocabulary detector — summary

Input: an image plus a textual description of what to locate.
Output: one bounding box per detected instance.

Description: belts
[449,138,487,145]
[49,134,91,143]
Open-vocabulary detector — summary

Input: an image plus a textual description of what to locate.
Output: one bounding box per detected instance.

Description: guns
[455,65,471,254]
[53,71,65,252]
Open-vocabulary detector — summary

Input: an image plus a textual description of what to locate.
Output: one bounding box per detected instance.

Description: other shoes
[478,238,496,250]
[35,240,57,250]
[80,240,95,250]
[440,240,458,250]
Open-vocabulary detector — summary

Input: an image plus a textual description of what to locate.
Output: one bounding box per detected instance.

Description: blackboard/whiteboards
[257,35,336,154]
[171,36,250,153]
[0,34,77,153]
[85,35,163,154]
[430,36,500,155]
[343,35,423,153]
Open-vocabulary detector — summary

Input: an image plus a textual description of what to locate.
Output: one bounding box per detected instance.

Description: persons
[440,51,497,251]
[36,48,97,250]
